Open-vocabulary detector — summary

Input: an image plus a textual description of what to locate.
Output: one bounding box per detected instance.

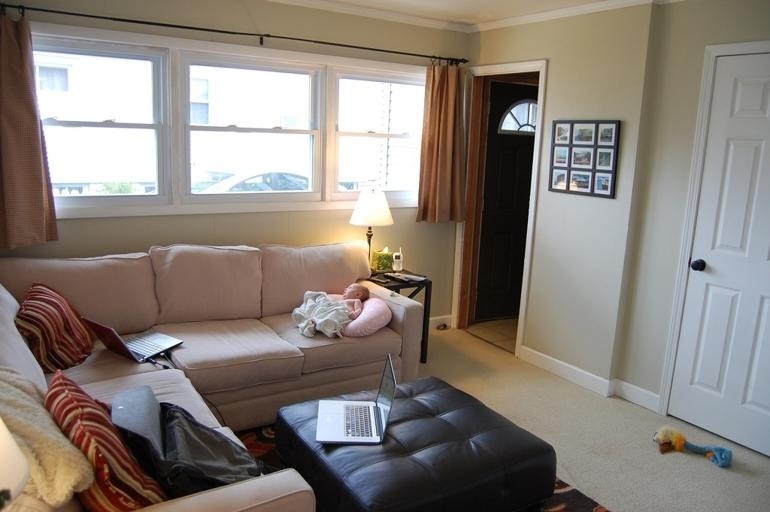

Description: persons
[291,279,369,336]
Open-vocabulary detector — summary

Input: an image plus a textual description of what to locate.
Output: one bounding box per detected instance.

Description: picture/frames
[548,119,620,199]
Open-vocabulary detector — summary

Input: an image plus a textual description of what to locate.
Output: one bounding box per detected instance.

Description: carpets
[235,422,613,512]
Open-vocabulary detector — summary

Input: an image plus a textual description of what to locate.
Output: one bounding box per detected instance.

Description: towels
[292,290,352,339]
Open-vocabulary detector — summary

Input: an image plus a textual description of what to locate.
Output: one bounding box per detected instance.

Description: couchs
[45,278,424,512]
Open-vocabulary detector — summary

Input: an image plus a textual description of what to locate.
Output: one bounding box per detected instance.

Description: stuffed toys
[652,426,734,467]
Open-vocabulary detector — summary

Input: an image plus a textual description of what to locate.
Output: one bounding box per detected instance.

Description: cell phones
[371,276,390,284]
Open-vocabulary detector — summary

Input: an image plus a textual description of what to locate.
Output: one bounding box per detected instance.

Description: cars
[146,167,358,194]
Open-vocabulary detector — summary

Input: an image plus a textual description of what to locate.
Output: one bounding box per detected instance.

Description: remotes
[384,273,409,282]
[403,273,426,282]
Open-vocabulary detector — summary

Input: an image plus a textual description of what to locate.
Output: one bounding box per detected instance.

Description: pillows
[326,293,393,335]
[14,282,96,373]
[150,243,262,322]
[0,253,160,334]
[0,421,82,512]
[43,367,167,512]
[259,243,371,318]
[0,284,48,398]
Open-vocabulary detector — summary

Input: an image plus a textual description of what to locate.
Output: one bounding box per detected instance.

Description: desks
[372,270,432,364]
[275,376,556,512]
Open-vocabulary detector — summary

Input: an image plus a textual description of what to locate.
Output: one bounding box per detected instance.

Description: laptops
[80,316,183,363]
[315,353,398,444]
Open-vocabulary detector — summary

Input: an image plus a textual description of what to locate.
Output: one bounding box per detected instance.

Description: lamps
[348,188,395,260]
